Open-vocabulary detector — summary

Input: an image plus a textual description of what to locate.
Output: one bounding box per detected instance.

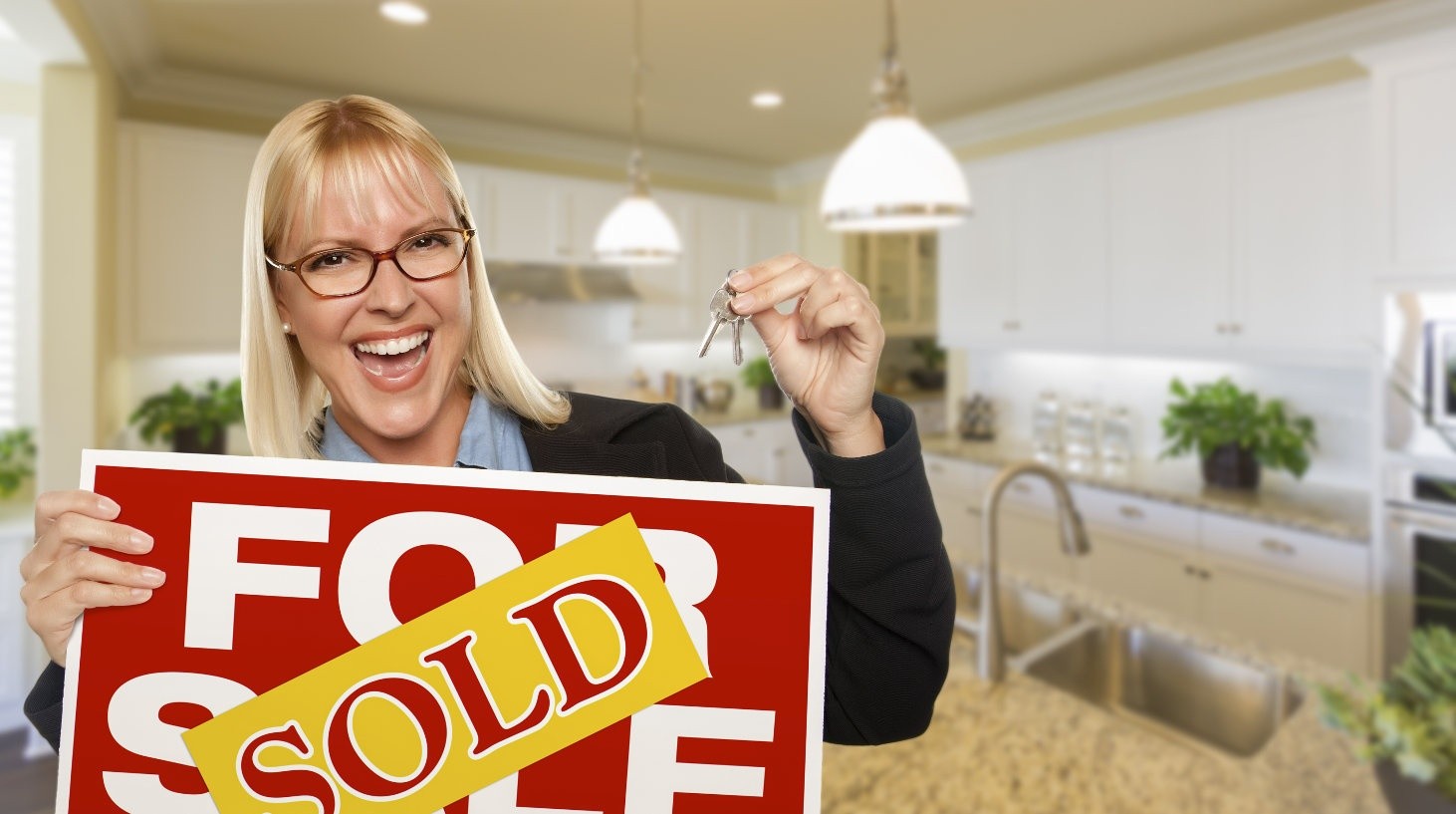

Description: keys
[700,289,743,354]
[720,281,751,364]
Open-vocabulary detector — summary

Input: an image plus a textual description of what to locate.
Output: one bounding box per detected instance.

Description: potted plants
[1150,368,1318,489]
[1320,622,1456,814]
[130,379,249,462]
[743,357,786,409]
[0,427,37,514]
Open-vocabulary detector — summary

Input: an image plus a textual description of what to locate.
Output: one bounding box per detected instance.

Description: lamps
[584,0,681,268]
[815,0,973,236]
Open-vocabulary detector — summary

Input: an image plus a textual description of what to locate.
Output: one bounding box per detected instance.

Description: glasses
[264,214,474,298]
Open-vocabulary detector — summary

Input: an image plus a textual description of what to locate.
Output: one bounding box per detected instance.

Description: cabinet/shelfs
[1075,522,1368,687]
[929,488,1055,595]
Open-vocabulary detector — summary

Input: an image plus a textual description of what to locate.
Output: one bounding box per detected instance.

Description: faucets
[976,462,1096,689]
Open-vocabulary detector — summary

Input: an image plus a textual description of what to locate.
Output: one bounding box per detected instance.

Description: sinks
[947,562,1098,674]
[1019,614,1304,764]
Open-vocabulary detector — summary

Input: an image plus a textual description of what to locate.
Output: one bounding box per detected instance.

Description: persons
[28,94,958,763]
